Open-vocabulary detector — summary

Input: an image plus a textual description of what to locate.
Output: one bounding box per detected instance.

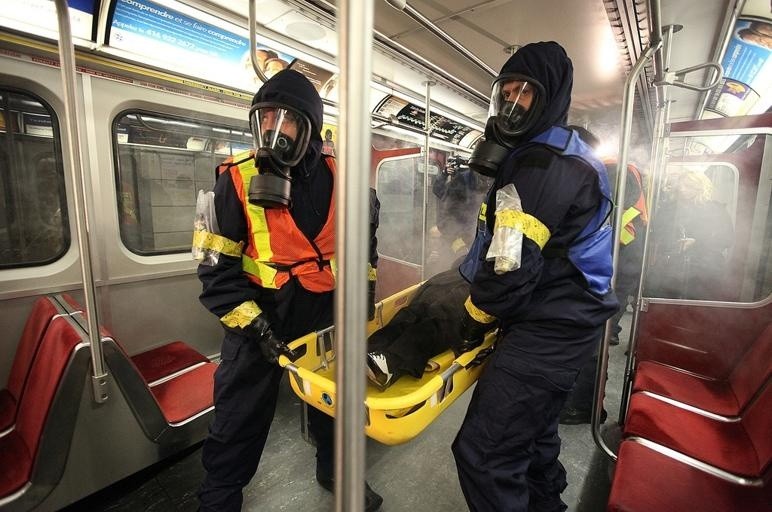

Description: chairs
[0,293,93,511]
[63,292,220,453]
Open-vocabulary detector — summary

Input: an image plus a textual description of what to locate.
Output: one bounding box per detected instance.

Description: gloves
[256,328,297,368]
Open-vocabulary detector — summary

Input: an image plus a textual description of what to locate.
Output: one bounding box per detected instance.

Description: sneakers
[314,468,383,512]
[365,347,395,392]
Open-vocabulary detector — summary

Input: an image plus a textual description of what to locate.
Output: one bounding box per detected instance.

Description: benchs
[606,299,770,512]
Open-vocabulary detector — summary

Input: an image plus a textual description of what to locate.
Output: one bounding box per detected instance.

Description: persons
[558,151,734,425]
[191,68,383,512]
[432,161,495,276]
[363,260,500,395]
[451,40,619,512]
[320,129,335,157]
